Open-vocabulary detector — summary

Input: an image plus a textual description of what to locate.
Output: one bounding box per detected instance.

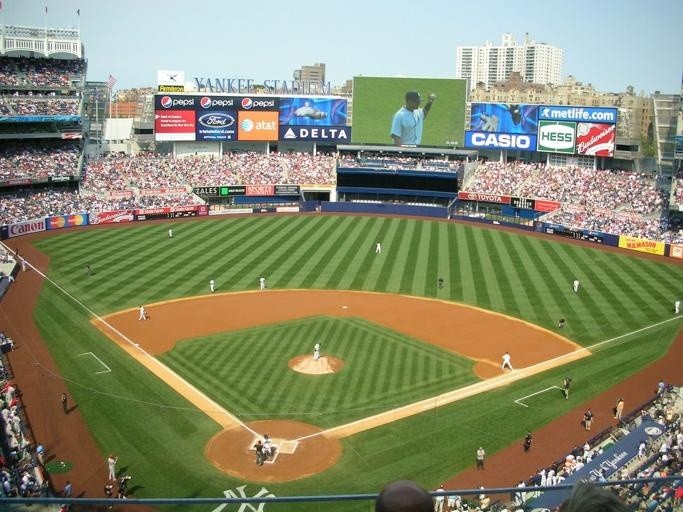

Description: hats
[405,91,424,104]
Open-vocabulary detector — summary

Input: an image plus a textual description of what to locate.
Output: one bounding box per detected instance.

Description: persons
[0,243,133,512]
[137,305,146,320]
[261,433,272,456]
[384,86,438,148]
[312,342,321,362]
[374,239,382,254]
[573,277,580,292]
[501,351,511,372]
[253,440,264,466]
[257,275,265,293]
[557,319,566,328]
[167,228,172,238]
[671,299,680,314]
[208,279,215,292]
